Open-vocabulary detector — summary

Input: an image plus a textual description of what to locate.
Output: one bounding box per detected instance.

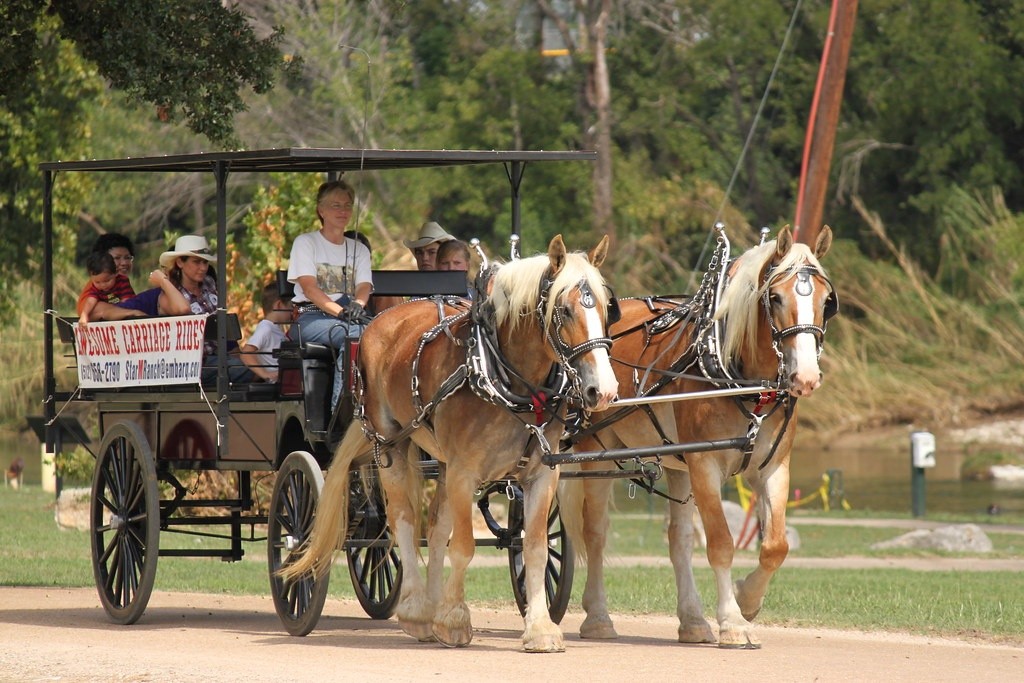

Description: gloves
[337,302,367,326]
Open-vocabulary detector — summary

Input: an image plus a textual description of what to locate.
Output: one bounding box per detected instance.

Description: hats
[159,235,217,271]
[403,221,458,260]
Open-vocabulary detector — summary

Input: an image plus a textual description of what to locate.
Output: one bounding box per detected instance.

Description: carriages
[40,149,838,656]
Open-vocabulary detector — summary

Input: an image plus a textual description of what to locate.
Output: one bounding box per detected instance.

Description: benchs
[76,313,279,394]
[274,270,343,359]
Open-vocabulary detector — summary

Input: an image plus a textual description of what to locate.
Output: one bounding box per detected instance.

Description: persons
[240,281,293,383]
[7,461,23,492]
[114,245,175,319]
[75,232,148,321]
[148,235,255,385]
[403,222,458,270]
[343,230,406,314]
[286,180,375,416]
[78,251,137,329]
[409,239,481,303]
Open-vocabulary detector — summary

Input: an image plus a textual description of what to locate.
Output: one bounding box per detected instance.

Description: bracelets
[160,276,168,286]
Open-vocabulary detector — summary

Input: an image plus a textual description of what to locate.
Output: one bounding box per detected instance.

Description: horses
[558,224,832,650]
[274,233,619,652]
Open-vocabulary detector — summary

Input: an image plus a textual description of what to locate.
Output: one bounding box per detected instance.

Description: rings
[150,272,153,275]
